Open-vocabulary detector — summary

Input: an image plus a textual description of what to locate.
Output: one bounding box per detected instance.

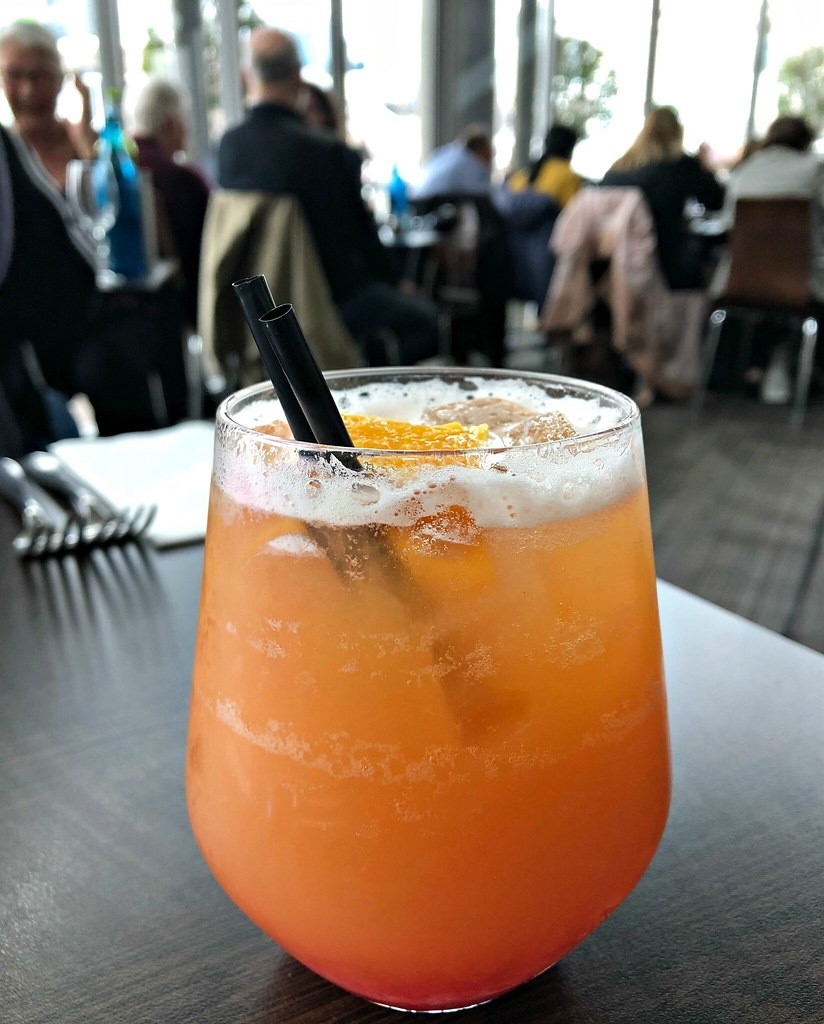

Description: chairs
[691,196,823,447]
[410,193,536,371]
[537,186,708,407]
[197,189,368,392]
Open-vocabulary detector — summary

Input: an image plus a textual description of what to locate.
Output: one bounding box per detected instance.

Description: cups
[185,367,669,1014]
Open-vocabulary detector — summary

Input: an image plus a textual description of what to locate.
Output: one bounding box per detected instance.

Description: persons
[217,28,452,367]
[0,20,824,433]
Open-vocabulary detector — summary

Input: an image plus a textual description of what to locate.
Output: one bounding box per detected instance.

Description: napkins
[46,417,214,549]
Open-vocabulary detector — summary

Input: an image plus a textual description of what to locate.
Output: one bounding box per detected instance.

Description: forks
[22,452,155,542]
[0,457,84,556]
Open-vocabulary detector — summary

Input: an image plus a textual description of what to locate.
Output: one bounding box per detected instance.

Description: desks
[0,487,824,1024]
